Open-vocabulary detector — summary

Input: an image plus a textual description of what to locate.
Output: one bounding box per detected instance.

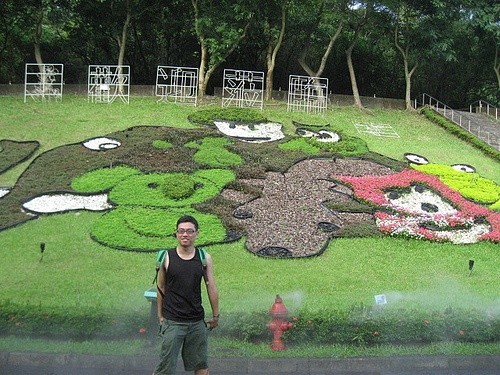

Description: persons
[153,214,220,375]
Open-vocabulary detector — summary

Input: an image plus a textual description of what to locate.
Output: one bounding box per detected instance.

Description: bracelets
[213,313,220,318]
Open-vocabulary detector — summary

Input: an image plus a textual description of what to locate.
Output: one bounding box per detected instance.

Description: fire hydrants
[265,293,296,351]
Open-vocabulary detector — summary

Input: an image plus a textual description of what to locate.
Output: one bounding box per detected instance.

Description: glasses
[177,228,196,234]
